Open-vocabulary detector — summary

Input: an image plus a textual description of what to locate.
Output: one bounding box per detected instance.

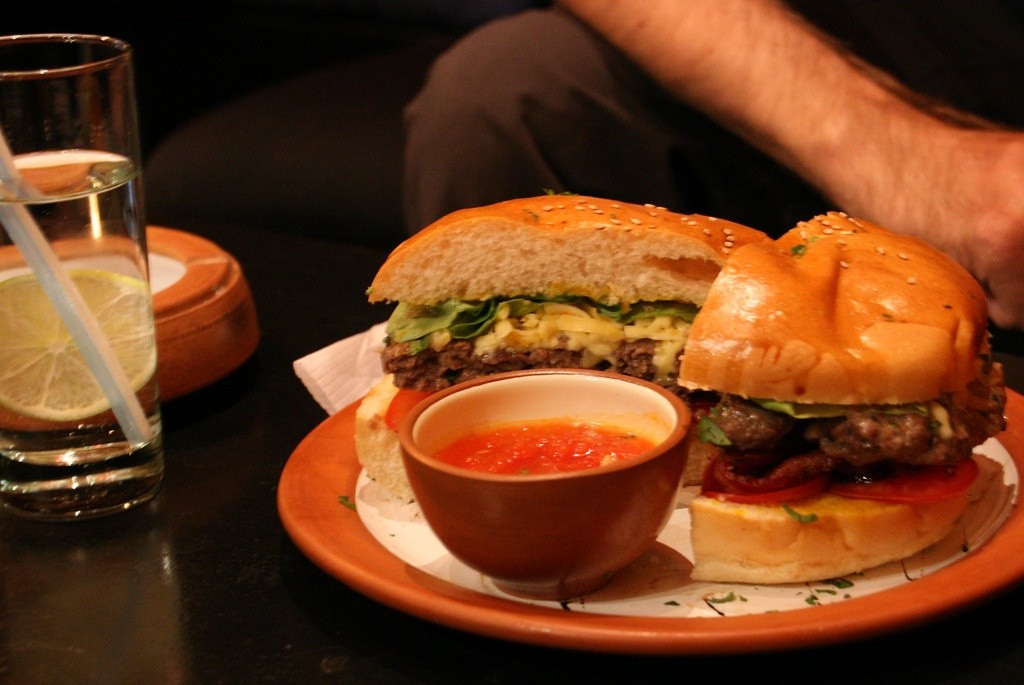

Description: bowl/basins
[398,366,693,600]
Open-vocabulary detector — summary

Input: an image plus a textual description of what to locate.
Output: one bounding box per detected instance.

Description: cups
[0,28,166,523]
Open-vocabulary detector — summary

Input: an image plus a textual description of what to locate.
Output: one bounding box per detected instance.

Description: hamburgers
[352,187,777,501]
[675,212,1005,582]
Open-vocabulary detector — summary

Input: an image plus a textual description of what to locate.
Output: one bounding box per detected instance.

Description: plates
[279,362,1023,651]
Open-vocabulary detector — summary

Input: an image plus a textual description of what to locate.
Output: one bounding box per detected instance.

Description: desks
[0,228,1023,684]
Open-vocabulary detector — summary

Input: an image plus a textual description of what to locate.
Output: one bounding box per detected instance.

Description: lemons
[0,267,157,422]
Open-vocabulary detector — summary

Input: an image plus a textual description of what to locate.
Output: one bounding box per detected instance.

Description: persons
[400,2,1024,329]
